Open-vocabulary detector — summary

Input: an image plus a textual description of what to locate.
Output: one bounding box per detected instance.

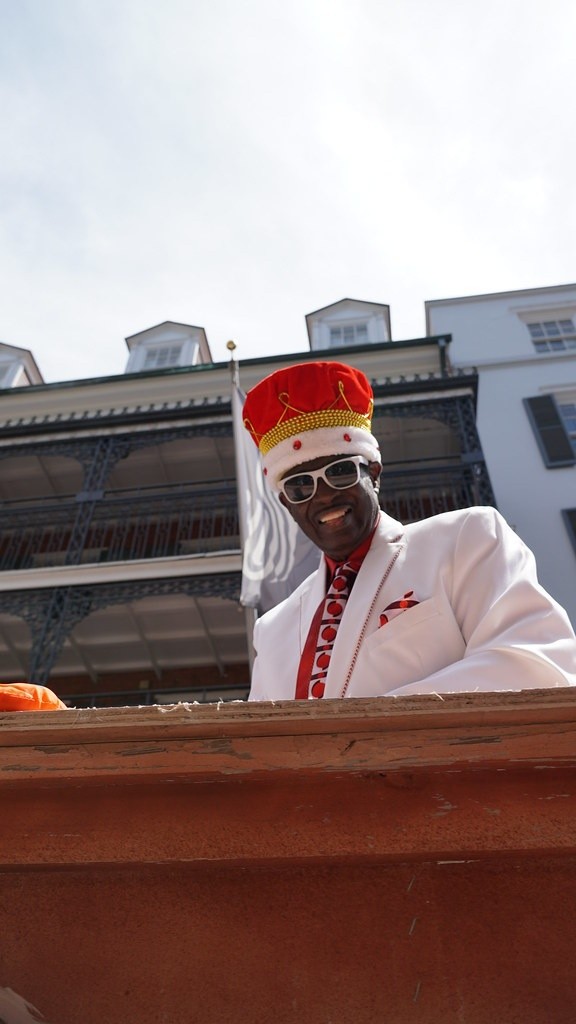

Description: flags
[231,363,323,614]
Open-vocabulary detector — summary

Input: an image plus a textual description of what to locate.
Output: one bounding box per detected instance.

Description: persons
[242,361,576,701]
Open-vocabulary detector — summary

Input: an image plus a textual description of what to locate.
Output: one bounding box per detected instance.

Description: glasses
[275,454,370,504]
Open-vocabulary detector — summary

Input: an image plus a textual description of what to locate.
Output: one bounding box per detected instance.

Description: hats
[243,361,382,494]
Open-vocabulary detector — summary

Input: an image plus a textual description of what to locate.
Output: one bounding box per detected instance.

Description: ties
[307,561,359,701]
